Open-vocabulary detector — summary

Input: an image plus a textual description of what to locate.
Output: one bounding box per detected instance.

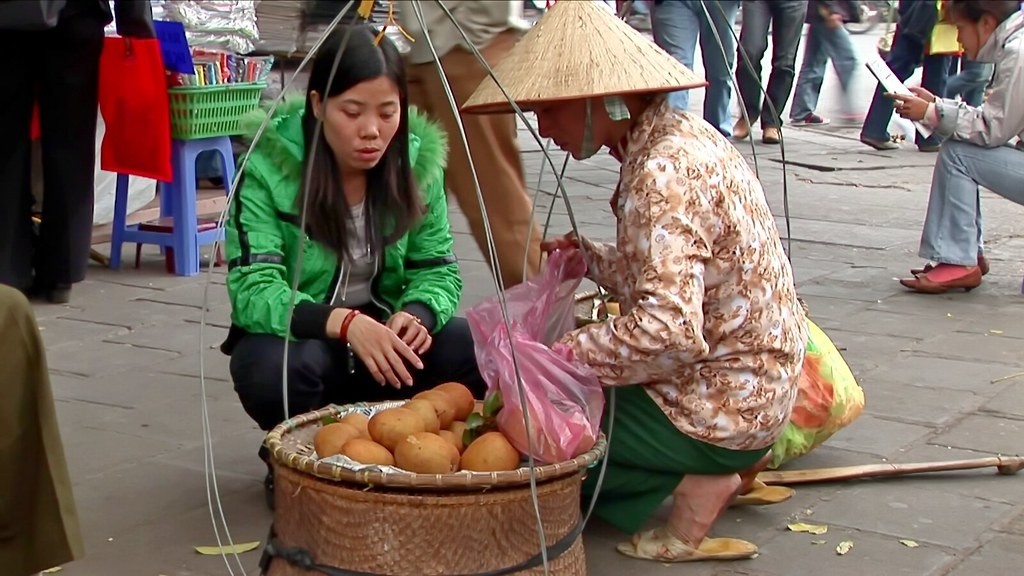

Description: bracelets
[340,309,361,344]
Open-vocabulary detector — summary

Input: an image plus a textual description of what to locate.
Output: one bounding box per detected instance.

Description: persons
[221,25,489,498]
[616,0,995,152]
[0,0,156,303]
[461,0,807,562]
[398,0,550,291]
[883,0,1024,293]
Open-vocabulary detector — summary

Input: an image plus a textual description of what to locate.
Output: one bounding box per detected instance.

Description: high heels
[900,257,989,294]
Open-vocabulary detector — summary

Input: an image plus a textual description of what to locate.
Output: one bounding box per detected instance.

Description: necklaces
[339,193,367,307]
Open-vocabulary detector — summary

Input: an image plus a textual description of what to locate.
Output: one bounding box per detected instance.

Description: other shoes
[919,145,940,152]
[46,284,73,303]
[733,115,758,139]
[762,127,783,144]
[861,134,898,149]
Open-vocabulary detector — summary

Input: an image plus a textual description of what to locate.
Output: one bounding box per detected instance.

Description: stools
[109,137,236,275]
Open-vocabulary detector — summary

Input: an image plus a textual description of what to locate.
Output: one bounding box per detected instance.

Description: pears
[597,301,621,318]
[314,380,598,476]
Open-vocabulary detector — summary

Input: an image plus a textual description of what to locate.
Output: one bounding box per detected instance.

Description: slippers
[789,114,830,126]
[727,477,795,506]
[615,524,758,563]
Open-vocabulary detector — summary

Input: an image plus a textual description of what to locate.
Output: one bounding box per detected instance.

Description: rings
[900,103,904,110]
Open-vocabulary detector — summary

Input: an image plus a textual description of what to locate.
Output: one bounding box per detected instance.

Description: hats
[459,0,710,114]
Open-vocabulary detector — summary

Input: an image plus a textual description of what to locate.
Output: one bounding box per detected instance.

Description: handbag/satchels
[768,313,864,468]
[464,244,605,463]
[929,21,962,55]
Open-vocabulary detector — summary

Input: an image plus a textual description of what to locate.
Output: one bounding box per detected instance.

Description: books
[865,54,934,139]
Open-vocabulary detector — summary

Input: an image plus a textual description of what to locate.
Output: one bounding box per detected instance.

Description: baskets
[168,81,268,139]
[258,460,607,576]
